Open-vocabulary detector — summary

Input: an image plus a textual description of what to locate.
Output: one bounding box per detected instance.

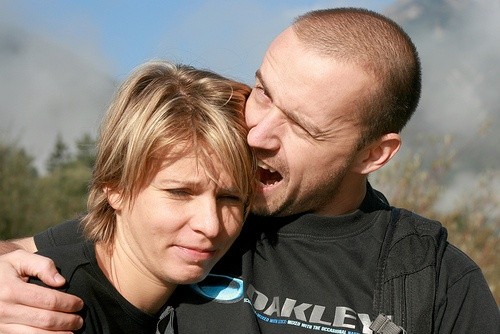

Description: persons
[0,7,500,334]
[25,60,262,334]
